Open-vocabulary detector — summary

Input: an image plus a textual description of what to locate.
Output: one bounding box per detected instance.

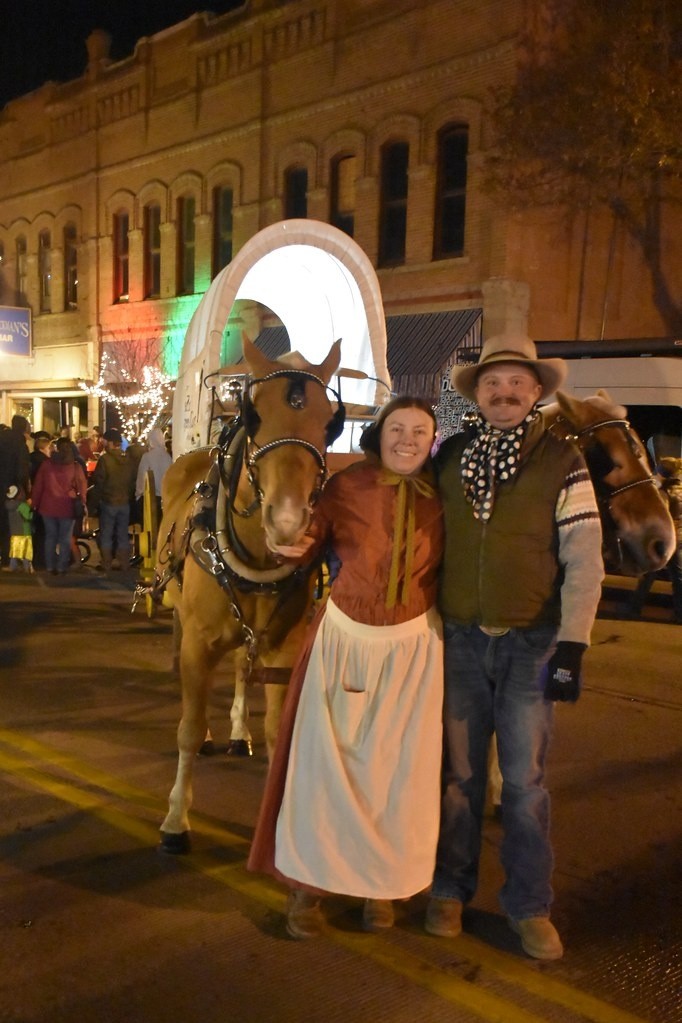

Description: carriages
[136,219,675,869]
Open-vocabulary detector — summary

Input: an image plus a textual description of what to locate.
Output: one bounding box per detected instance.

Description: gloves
[542,642,587,704]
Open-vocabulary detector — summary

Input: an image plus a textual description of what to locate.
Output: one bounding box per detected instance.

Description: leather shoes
[359,894,397,930]
[284,886,327,941]
[422,893,464,937]
[508,912,564,960]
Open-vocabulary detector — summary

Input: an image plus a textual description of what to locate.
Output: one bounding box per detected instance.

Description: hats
[449,330,567,406]
[5,484,26,501]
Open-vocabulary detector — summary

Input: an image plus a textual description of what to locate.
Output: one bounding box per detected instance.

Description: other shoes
[1,555,130,576]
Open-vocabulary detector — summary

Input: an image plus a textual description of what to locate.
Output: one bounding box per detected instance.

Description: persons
[0,414,172,576]
[613,457,681,622]
[248,397,445,939]
[424,334,605,960]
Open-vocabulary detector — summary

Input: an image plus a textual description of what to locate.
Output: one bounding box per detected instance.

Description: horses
[153,326,347,855]
[487,384,679,819]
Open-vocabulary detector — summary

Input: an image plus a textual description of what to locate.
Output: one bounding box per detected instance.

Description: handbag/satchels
[76,503,91,535]
